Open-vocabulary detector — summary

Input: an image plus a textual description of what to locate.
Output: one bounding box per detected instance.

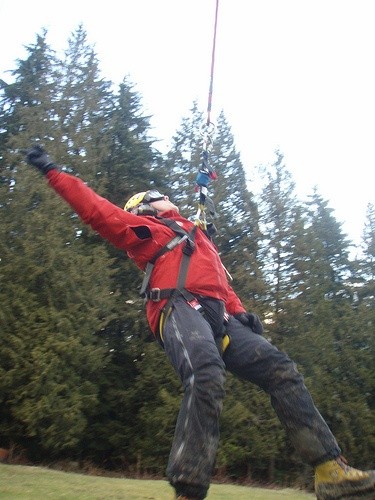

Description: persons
[18,143,375,500]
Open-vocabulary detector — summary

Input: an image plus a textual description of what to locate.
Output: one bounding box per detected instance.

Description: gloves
[234,312,264,335]
[19,145,58,174]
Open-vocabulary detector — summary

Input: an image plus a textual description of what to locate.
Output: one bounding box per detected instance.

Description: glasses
[142,190,164,203]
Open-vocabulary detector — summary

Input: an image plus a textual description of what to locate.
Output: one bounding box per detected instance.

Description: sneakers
[314,454,375,500]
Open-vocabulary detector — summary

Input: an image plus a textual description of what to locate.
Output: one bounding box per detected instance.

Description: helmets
[124,192,146,212]
